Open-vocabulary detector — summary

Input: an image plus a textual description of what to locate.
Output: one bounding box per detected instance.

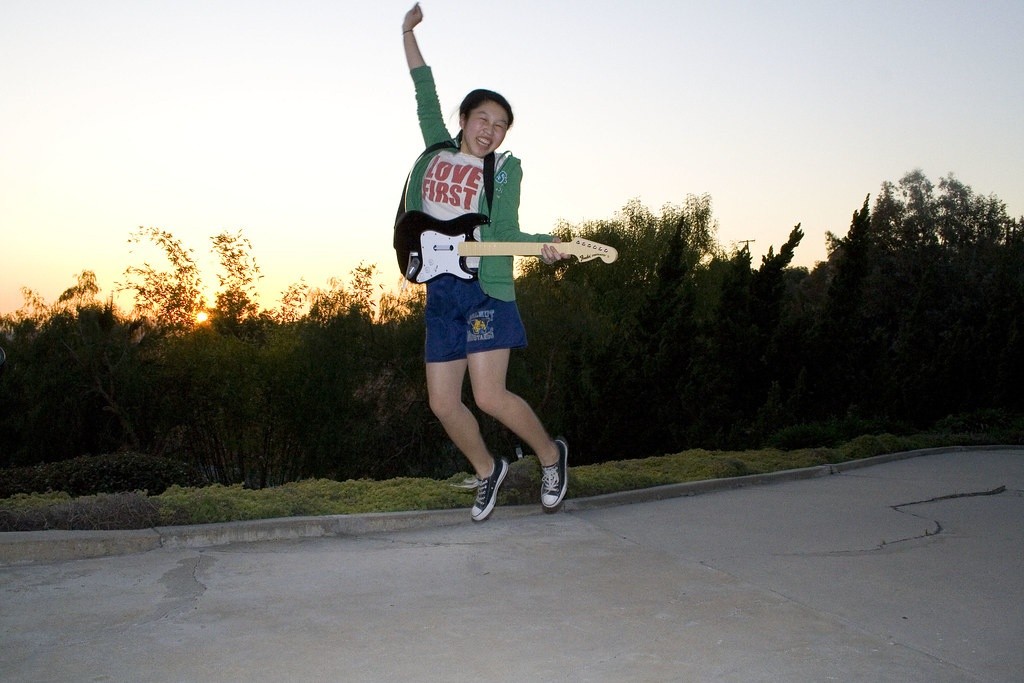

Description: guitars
[393,210,618,286]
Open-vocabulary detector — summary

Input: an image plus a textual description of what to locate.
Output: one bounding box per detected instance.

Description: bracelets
[403,29,413,35]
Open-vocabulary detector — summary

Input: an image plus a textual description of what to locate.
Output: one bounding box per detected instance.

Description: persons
[393,2,571,523]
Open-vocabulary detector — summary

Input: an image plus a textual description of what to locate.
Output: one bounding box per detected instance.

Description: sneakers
[471,459,509,521]
[540,439,568,508]
